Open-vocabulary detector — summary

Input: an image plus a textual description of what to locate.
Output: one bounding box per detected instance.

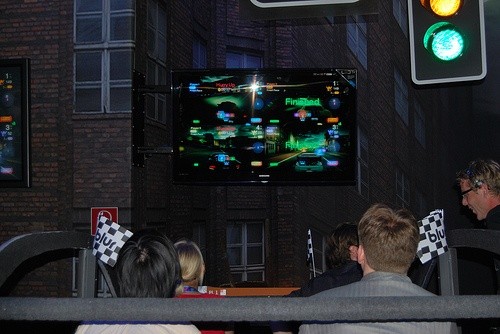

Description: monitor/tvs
[0,58,32,188]
[171,67,358,186]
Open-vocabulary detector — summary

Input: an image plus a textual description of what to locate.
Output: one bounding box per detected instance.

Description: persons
[169,239,234,334]
[455,160,500,334]
[284,221,363,334]
[75,229,201,334]
[298,206,459,334]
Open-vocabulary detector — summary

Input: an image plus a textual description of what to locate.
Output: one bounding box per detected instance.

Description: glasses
[458,185,478,199]
